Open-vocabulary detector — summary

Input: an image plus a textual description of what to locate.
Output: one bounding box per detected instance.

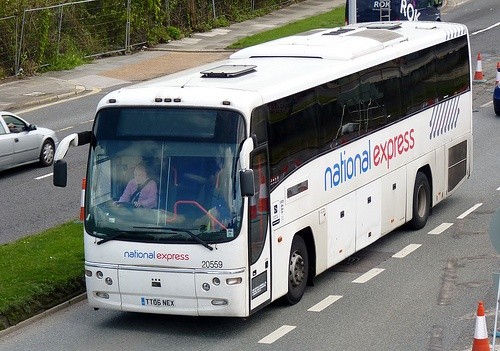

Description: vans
[344,1,444,24]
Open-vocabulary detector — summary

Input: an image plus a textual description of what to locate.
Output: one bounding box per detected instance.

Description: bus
[52,21,474,321]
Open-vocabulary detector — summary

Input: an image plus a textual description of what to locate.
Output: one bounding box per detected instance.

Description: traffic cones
[471,52,487,83]
[250,193,262,224]
[471,300,490,351]
[256,177,268,216]
[74,179,85,223]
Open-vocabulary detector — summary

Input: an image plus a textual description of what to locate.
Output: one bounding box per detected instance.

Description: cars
[0,111,59,173]
